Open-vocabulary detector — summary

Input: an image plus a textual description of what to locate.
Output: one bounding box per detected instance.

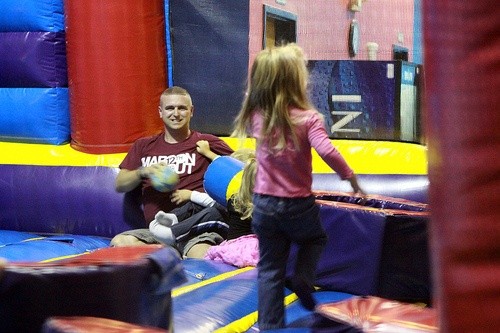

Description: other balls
[151,165,179,193]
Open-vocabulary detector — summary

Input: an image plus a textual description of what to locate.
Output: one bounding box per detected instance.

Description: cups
[366,42,378,60]
